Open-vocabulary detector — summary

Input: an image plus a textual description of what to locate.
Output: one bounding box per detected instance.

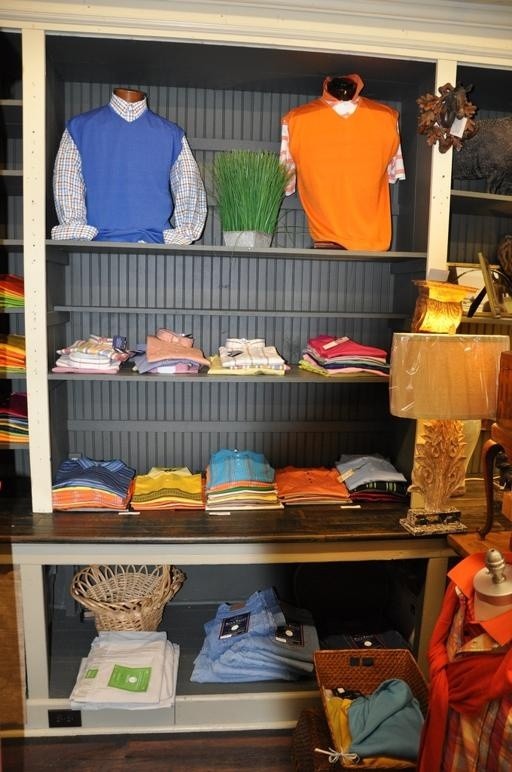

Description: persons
[422,550,512,771]
[52,87,208,240]
[280,75,406,249]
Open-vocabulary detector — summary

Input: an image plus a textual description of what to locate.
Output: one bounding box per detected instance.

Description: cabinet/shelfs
[0,514,512,742]
[2,26,511,514]
